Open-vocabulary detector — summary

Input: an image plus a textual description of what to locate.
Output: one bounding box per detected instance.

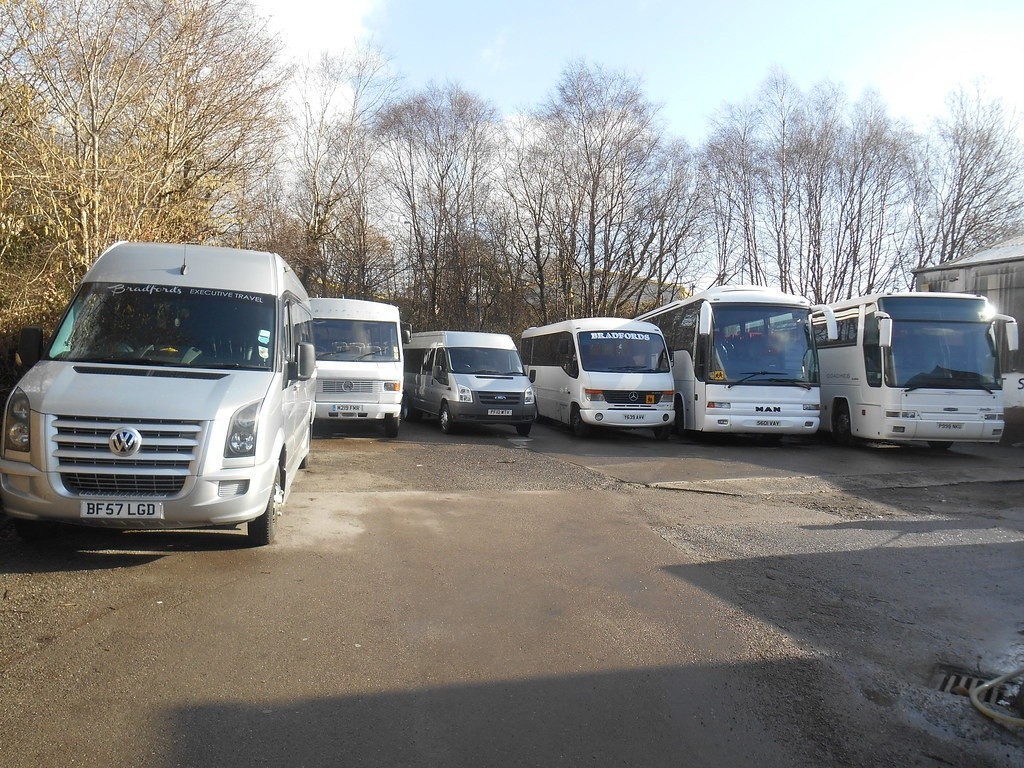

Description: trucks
[309,298,412,437]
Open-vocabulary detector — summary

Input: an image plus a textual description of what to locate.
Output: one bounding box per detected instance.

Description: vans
[402,330,537,436]
[0,238,317,546]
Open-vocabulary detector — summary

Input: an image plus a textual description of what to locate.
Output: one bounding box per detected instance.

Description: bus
[521,317,675,441]
[632,283,839,448]
[735,291,1019,450]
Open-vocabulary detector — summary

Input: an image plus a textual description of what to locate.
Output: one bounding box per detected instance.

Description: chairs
[332,341,382,356]
